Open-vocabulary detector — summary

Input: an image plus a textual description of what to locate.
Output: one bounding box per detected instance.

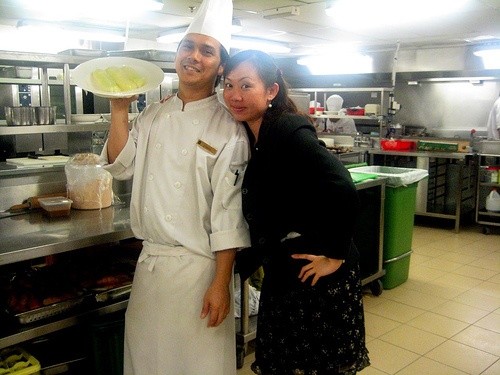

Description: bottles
[485,187,500,213]
[485,166,500,184]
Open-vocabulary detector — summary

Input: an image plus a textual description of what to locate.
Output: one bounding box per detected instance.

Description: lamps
[155,26,293,54]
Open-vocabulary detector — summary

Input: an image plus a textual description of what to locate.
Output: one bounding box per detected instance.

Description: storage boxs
[38,195,73,218]
[380,137,471,153]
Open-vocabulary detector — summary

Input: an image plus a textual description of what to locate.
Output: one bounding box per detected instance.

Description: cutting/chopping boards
[6,156,70,166]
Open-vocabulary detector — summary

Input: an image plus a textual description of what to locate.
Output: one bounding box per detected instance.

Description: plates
[72,56,165,98]
[62,114,102,124]
[102,113,135,122]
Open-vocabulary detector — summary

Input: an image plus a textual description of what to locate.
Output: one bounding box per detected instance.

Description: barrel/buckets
[65,162,112,210]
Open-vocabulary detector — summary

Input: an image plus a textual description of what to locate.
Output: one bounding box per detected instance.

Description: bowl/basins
[37,196,73,220]
[3,105,35,126]
[35,106,57,125]
[314,110,324,116]
[324,111,338,116]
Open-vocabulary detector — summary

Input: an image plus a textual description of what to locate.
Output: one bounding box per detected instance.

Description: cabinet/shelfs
[475,152,500,234]
[288,86,393,147]
[0,47,161,135]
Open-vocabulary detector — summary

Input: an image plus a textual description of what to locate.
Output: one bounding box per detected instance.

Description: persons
[325,93,357,135]
[221,49,372,375]
[486,91,500,140]
[98,19,252,374]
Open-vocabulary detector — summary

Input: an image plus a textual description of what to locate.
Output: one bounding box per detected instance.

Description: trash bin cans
[347,165,429,290]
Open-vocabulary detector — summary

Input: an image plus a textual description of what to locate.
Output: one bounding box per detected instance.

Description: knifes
[28,153,47,160]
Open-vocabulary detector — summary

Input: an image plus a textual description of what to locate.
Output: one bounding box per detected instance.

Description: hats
[184,0,233,55]
[326,95,343,111]
